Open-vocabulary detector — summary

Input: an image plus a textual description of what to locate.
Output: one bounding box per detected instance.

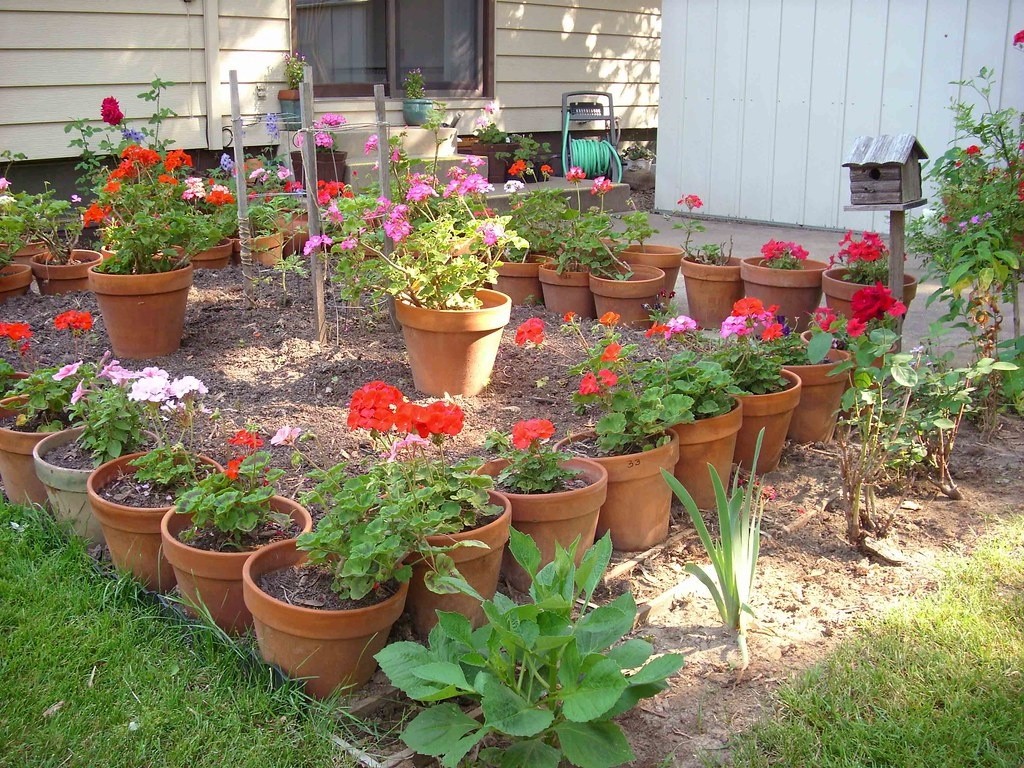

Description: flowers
[0,52,909,513]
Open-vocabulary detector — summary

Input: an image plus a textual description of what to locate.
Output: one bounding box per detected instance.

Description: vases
[0,88,920,698]
